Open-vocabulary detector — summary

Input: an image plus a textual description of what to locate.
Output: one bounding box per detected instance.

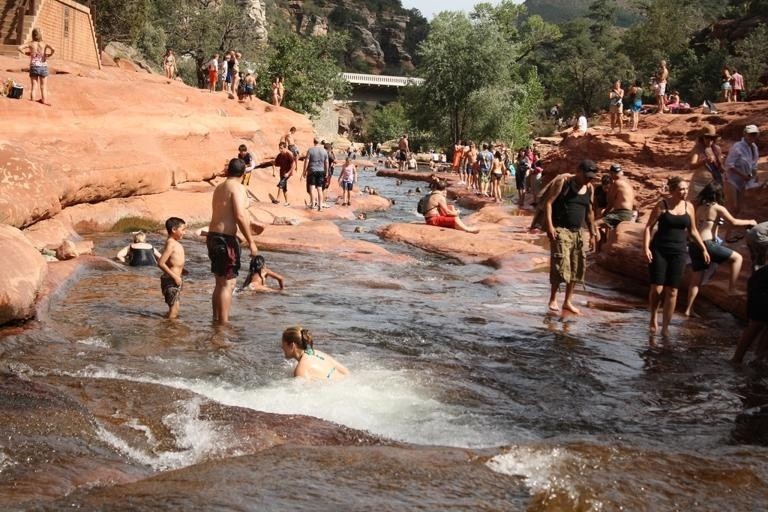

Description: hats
[610,164,622,172]
[744,125,760,133]
[700,124,719,136]
[578,159,597,179]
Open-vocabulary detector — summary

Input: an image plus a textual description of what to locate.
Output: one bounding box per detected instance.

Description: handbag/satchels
[7,86,23,99]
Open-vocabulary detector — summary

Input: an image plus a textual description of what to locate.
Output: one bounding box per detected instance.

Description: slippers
[725,230,744,242]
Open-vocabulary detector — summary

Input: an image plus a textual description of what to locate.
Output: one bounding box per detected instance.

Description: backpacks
[530,172,570,232]
[417,193,438,214]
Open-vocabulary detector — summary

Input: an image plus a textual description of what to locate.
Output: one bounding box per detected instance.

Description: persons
[279,126,300,171]
[300,137,329,212]
[271,141,296,208]
[684,181,760,318]
[163,48,176,80]
[270,77,280,107]
[279,325,352,381]
[423,180,480,235]
[642,177,710,344]
[627,78,646,132]
[607,79,626,136]
[277,76,286,102]
[729,69,745,102]
[724,124,763,243]
[721,70,732,104]
[206,157,258,322]
[542,160,600,315]
[588,174,613,253]
[650,59,670,116]
[322,130,544,219]
[237,143,256,186]
[721,220,768,374]
[157,216,187,321]
[206,48,256,102]
[18,26,56,104]
[586,163,635,257]
[667,90,680,113]
[545,101,590,137]
[687,123,742,245]
[242,254,285,293]
